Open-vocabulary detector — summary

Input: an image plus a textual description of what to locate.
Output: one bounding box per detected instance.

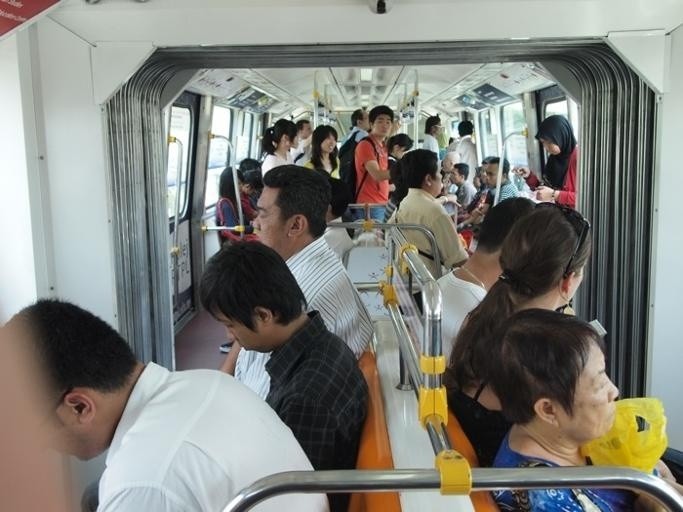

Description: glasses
[535,202,590,275]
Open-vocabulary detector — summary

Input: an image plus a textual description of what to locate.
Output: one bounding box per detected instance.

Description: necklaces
[460,261,484,290]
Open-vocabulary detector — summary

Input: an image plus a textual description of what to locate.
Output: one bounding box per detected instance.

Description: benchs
[218,221,682,512]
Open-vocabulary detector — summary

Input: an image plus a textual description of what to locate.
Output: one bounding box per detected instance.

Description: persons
[219,164,374,401]
[80,238,368,512]
[434,197,535,366]
[218,177,353,352]
[0,299,330,512]
[446,203,632,468]
[487,308,683,512]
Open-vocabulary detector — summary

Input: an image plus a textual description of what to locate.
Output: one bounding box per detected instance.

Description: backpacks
[337,136,379,205]
